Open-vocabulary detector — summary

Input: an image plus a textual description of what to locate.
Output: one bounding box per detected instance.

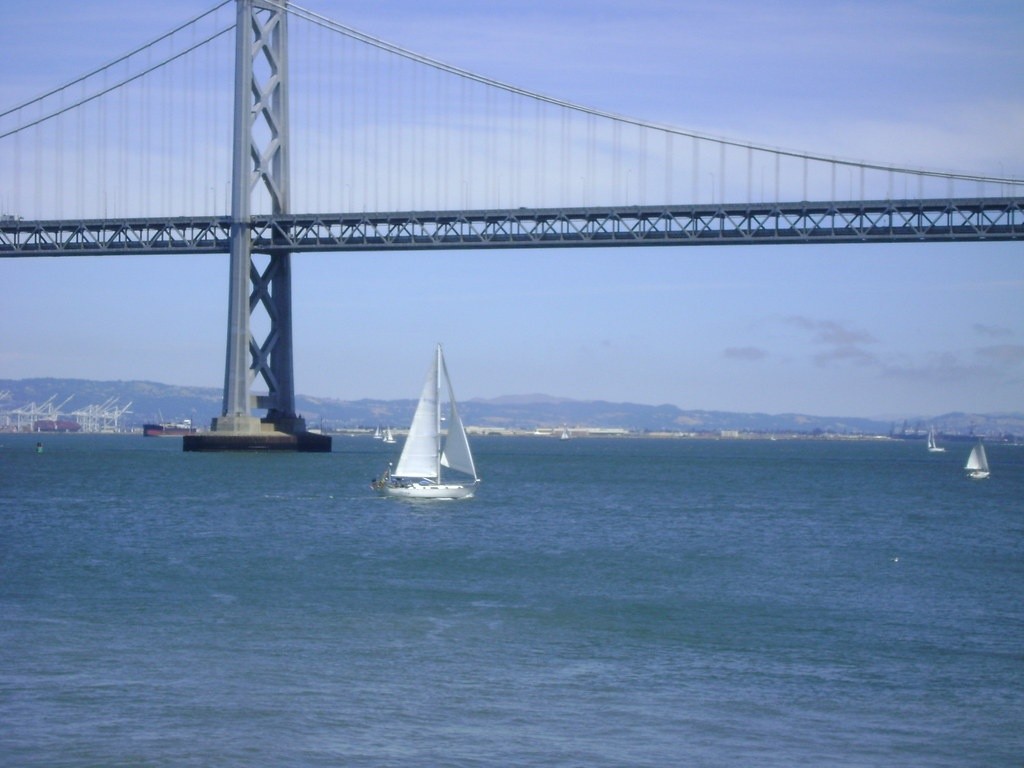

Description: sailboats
[374,423,397,443]
[963,436,991,480]
[927,425,945,453]
[370,341,481,499]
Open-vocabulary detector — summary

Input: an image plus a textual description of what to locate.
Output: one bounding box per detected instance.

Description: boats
[143,417,196,437]
